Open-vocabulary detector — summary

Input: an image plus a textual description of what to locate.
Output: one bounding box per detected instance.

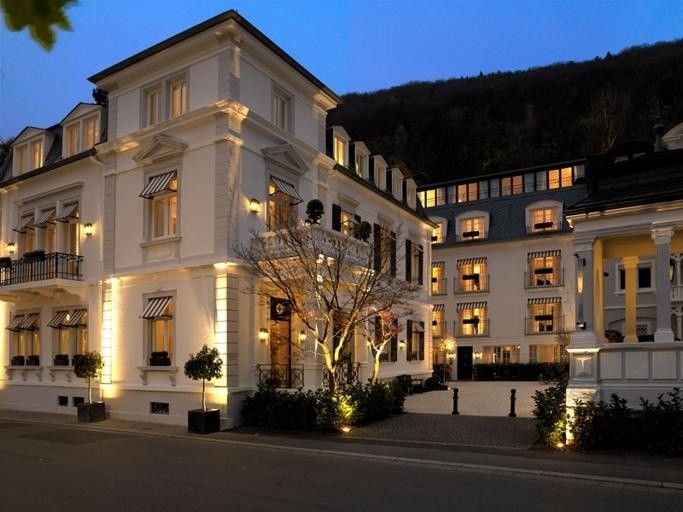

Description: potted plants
[77,350,106,421]
[186,347,224,436]
